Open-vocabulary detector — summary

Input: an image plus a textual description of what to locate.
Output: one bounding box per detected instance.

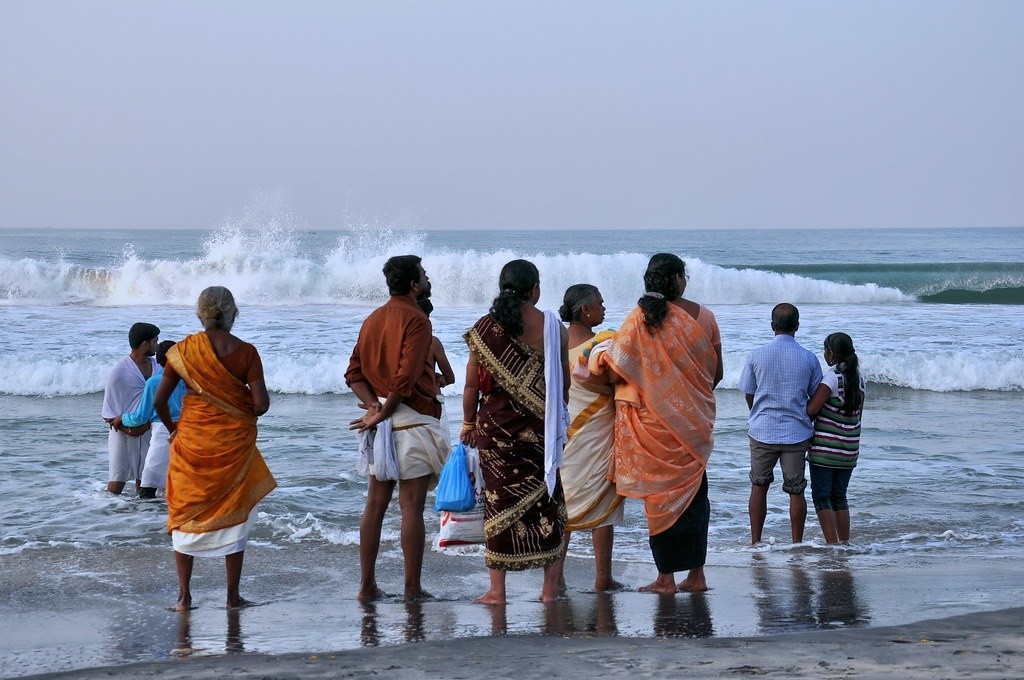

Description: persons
[459,259,571,605]
[344,255,442,602]
[417,296,455,494]
[105,340,189,499]
[558,284,632,593]
[153,286,270,611]
[739,303,823,545]
[807,332,866,546]
[101,322,160,495]
[601,253,723,594]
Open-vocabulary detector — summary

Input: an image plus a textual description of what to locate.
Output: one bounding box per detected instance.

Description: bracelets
[463,421,476,430]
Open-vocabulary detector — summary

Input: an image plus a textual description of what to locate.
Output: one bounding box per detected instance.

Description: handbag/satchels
[436,442,491,548]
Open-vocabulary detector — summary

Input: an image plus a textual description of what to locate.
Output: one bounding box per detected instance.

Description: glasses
[680,273,690,282]
